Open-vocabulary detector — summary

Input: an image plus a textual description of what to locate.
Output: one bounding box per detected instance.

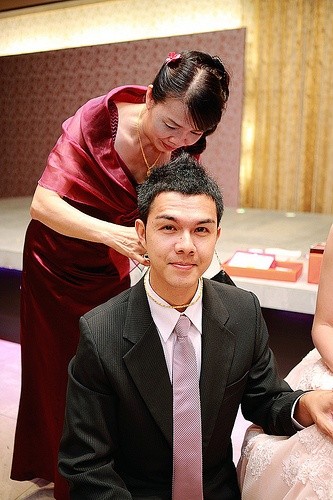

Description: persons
[237,223,333,500]
[11,50,231,500]
[56,153,333,500]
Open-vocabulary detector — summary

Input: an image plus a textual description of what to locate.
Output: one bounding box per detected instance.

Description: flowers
[165,52,181,65]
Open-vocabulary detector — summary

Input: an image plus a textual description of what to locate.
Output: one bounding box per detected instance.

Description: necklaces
[136,104,162,178]
[143,267,204,308]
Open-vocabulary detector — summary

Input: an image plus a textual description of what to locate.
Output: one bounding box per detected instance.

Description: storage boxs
[308,242,325,283]
[222,259,303,282]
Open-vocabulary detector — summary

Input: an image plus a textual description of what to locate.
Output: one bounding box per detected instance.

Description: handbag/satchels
[211,271,236,286]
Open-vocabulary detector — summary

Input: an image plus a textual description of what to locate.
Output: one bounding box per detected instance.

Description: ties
[173,314,205,500]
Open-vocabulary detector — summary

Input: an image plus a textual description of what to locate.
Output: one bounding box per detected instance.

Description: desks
[229,248,318,314]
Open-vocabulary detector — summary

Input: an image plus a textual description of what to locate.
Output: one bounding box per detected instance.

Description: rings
[144,253,149,261]
